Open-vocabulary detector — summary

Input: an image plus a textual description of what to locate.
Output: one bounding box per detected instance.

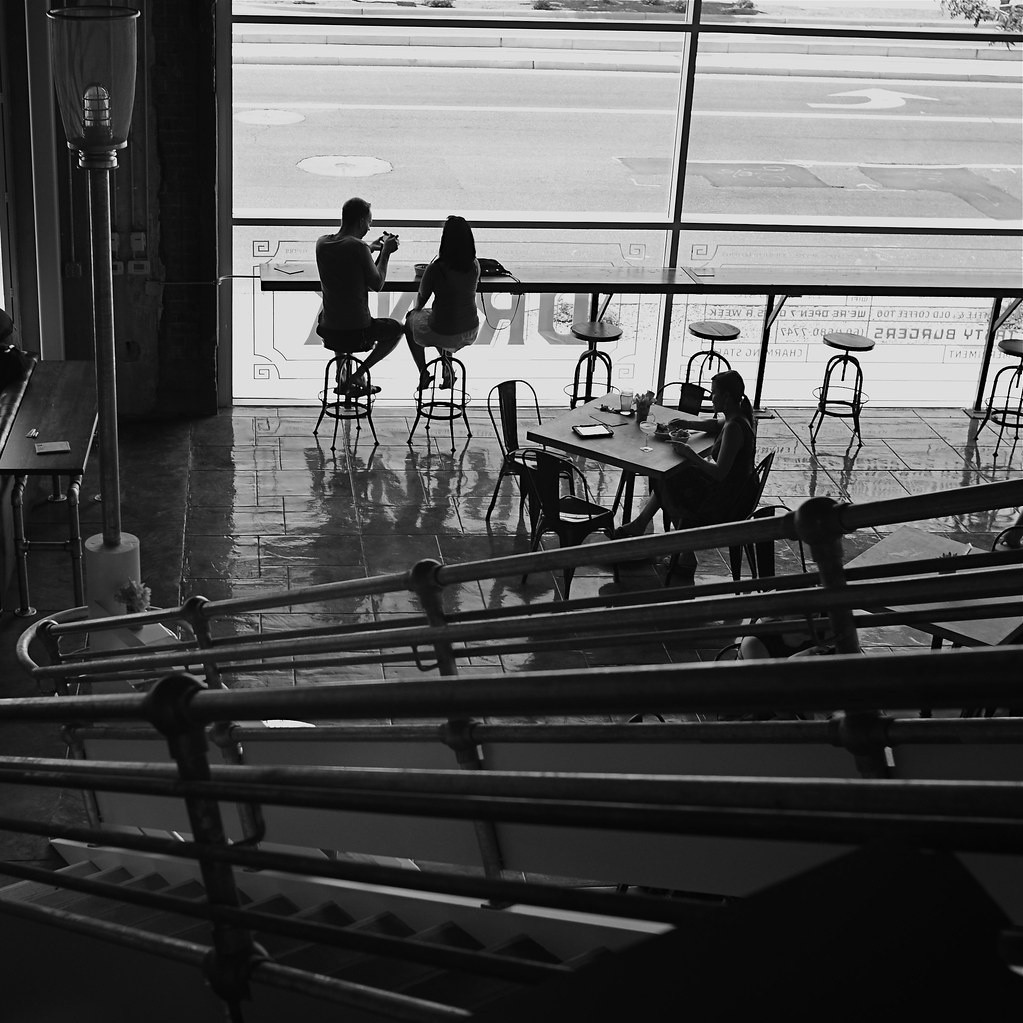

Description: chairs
[611,380,717,517]
[674,449,778,593]
[744,504,807,580]
[486,378,577,526]
[510,446,617,599]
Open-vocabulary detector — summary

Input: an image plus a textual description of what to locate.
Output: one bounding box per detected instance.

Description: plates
[653,429,674,440]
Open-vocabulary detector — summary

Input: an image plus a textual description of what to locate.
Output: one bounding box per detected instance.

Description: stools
[808,332,875,449]
[311,332,379,453]
[685,320,741,415]
[967,338,1023,459]
[562,318,624,410]
[398,343,473,453]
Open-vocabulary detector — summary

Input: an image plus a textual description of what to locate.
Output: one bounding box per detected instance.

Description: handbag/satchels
[478,257,507,276]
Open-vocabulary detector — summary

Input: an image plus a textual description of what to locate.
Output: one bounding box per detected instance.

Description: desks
[842,524,1023,649]
[0,356,107,615]
[524,390,720,525]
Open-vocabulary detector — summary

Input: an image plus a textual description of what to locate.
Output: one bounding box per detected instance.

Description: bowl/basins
[414,263,428,277]
[669,429,691,446]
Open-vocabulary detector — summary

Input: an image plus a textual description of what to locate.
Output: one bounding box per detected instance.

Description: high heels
[605,525,633,541]
[661,555,696,576]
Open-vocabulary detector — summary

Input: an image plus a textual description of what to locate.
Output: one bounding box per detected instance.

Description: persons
[315,197,404,394]
[615,370,757,568]
[735,585,830,723]
[404,216,481,392]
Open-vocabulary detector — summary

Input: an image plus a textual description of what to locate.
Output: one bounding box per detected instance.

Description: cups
[620,391,634,411]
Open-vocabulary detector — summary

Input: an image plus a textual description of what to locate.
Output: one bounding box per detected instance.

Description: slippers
[348,385,382,398]
[439,377,458,389]
[332,387,348,395]
[417,375,435,391]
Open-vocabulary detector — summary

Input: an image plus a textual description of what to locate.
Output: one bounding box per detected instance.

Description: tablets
[571,423,614,438]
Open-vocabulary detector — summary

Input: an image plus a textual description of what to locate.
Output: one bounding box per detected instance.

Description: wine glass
[639,421,658,452]
